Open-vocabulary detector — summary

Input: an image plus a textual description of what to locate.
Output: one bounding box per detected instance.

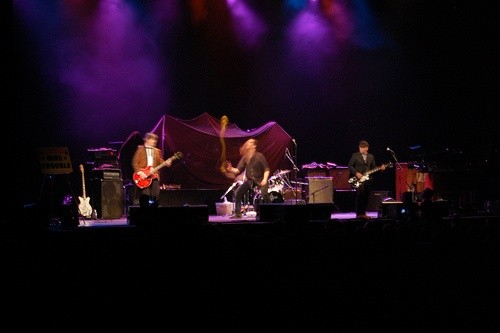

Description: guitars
[346,163,392,189]
[77,164,94,217]
[133,152,184,189]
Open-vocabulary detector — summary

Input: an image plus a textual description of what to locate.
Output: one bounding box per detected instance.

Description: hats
[359,141,369,147]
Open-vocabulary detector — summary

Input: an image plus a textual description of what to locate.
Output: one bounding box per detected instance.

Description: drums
[252,191,284,213]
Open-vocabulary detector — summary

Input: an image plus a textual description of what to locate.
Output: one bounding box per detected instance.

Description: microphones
[386,147,395,155]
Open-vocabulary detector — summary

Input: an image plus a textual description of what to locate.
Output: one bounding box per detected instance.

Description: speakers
[256,202,333,222]
[129,205,209,226]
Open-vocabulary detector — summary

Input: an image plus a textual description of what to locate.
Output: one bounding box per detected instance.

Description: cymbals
[268,171,292,181]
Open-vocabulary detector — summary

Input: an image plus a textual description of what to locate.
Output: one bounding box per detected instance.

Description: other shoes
[356,215,371,219]
[229,214,242,219]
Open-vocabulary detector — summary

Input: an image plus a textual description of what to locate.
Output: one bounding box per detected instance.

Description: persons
[132,133,173,208]
[348,140,386,219]
[225,138,270,219]
[139,217,499,333]
[224,147,250,217]
